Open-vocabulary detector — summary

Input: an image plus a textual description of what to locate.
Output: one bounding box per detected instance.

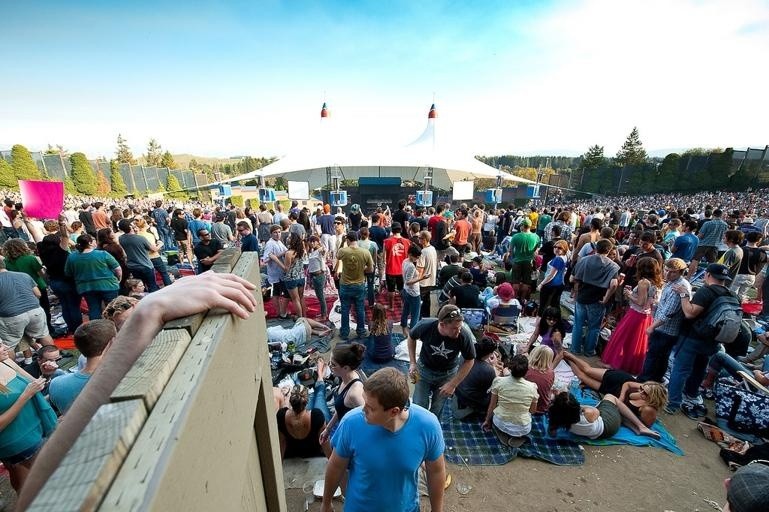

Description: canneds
[624,284,632,291]
[618,272,626,285]
[629,254,638,264]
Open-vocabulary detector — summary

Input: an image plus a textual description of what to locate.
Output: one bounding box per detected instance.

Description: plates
[312,479,341,500]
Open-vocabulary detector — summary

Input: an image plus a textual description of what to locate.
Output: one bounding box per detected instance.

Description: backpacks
[694,293,743,345]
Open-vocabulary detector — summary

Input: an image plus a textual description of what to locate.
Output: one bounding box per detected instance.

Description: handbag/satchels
[714,379,769,436]
[15,374,59,434]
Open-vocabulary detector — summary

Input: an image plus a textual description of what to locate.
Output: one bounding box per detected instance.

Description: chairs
[461,307,485,336]
[733,368,768,396]
[436,273,451,304]
[488,304,521,336]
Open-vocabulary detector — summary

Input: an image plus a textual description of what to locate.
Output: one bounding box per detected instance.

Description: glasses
[333,222,343,225]
[237,228,246,232]
[201,233,210,237]
[46,356,63,364]
[553,245,563,250]
[438,309,462,322]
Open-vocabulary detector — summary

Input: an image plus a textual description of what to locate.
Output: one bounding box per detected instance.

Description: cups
[303,481,317,504]
[618,254,637,293]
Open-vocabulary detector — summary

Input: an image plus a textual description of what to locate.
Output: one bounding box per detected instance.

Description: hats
[516,210,524,216]
[351,203,361,213]
[297,369,315,386]
[391,222,401,229]
[742,216,754,224]
[706,264,733,282]
[521,219,532,226]
[497,282,514,300]
[725,460,769,511]
[345,231,357,239]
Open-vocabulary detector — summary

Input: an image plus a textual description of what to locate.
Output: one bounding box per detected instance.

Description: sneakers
[279,311,294,319]
[491,422,525,448]
[664,390,708,421]
